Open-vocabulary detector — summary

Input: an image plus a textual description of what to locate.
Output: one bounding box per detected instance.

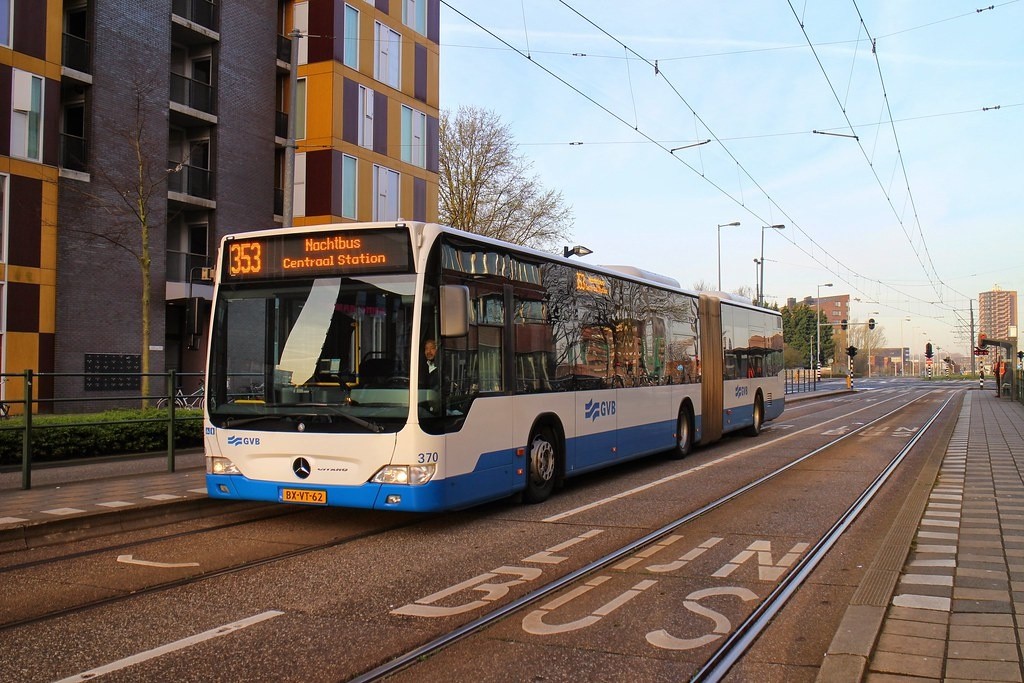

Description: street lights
[918,332,951,381]
[759,224,786,307]
[901,318,911,378]
[816,283,834,382]
[709,221,741,284]
[866,312,880,379]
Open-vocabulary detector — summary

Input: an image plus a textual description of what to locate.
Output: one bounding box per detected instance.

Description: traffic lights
[978,332,988,350]
[925,344,934,359]
[845,346,858,357]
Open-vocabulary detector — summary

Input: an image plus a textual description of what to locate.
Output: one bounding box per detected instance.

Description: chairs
[359,351,403,388]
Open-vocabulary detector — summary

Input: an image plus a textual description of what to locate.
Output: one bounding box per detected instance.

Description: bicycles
[228,379,266,403]
[0,377,12,421]
[155,371,219,415]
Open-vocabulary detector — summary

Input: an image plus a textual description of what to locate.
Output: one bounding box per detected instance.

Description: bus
[177,215,788,519]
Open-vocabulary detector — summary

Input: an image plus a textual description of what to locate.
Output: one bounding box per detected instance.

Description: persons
[418,339,447,390]
